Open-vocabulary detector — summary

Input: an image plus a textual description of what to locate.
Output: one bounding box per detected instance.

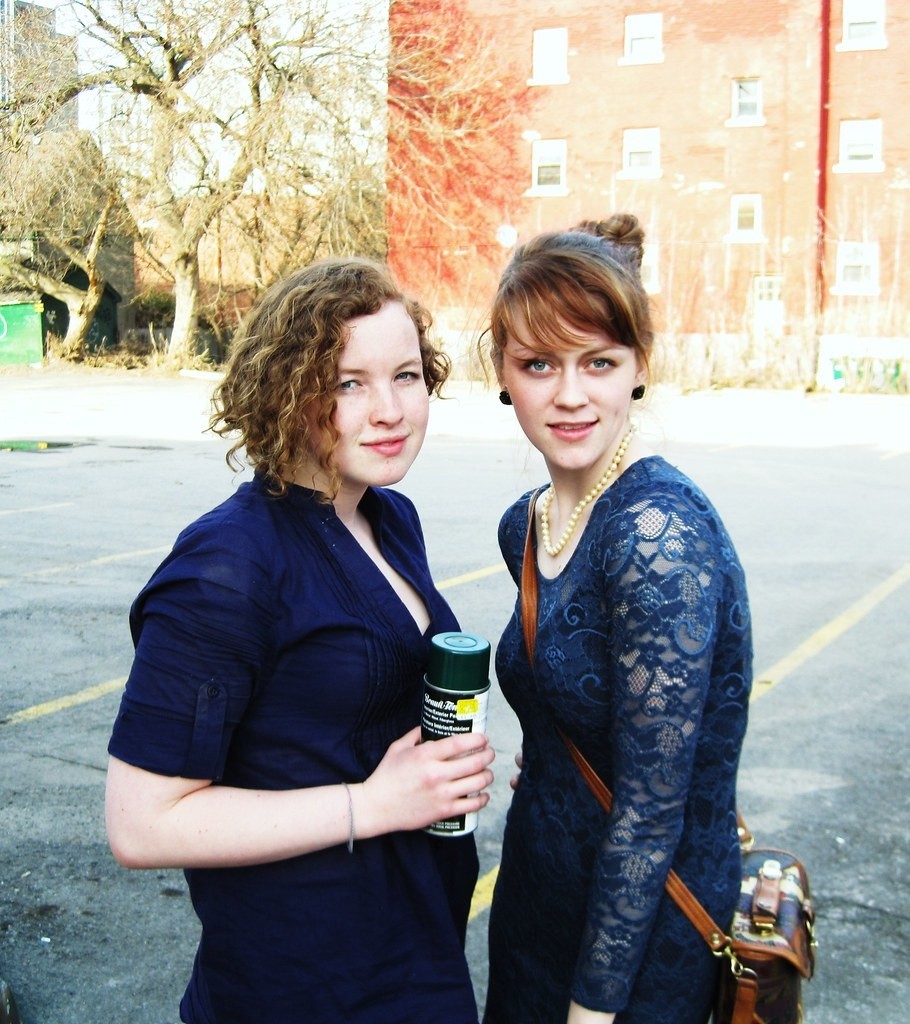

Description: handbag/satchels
[712,847,818,1024]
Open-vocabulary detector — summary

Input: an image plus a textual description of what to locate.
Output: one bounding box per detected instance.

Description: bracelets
[341,781,356,854]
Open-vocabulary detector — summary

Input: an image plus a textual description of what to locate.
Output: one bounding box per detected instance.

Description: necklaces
[540,424,640,557]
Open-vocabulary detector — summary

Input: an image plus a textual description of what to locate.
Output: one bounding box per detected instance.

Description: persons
[484,215,755,1024]
[101,259,494,1024]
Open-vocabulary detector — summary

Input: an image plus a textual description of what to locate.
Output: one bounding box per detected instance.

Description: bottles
[422,632,492,837]
